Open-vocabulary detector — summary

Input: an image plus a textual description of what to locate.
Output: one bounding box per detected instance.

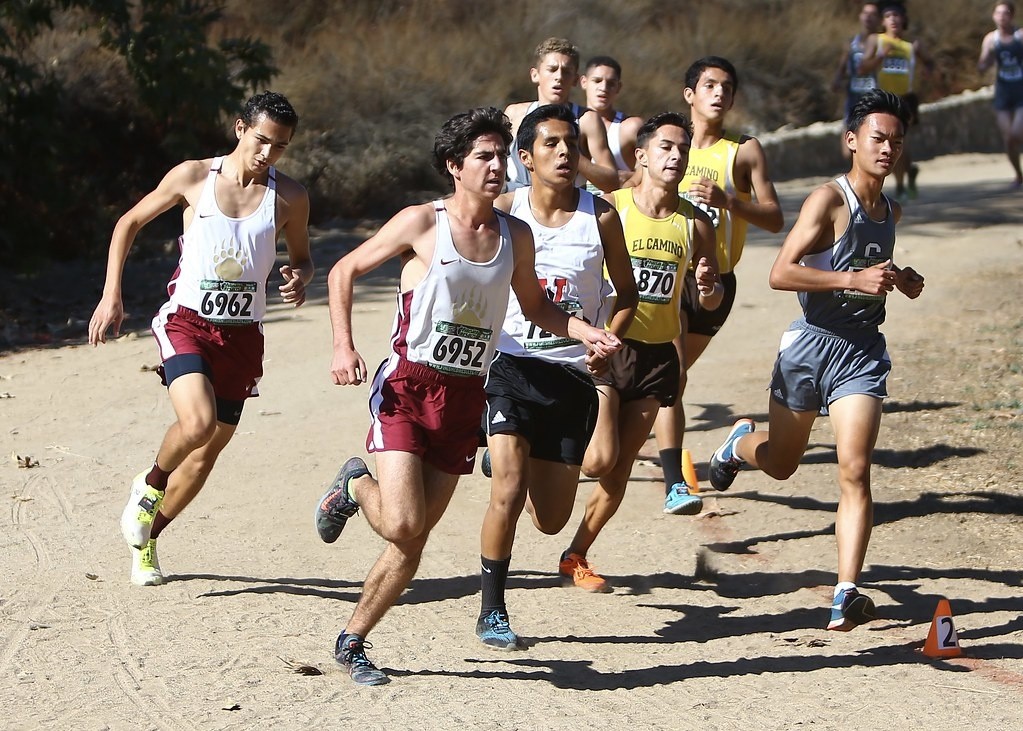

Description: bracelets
[700,286,715,297]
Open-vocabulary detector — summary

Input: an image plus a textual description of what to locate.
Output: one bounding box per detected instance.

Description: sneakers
[334,629,388,685]
[315,456,372,542]
[663,481,702,515]
[119,466,164,549]
[708,418,755,491]
[126,538,162,586]
[559,549,608,591]
[475,610,516,651]
[482,448,494,478]
[827,587,874,632]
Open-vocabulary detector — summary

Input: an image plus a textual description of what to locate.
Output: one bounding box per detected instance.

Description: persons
[708,87,925,632]
[837,0,882,167]
[977,0,1023,188]
[501,36,620,196]
[87,92,314,586]
[313,105,621,686]
[474,105,639,650]
[580,55,645,196]
[856,0,923,203]
[653,55,785,516]
[559,109,724,594]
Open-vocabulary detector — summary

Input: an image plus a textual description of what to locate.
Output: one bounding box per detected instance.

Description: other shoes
[907,163,918,188]
[892,185,904,204]
